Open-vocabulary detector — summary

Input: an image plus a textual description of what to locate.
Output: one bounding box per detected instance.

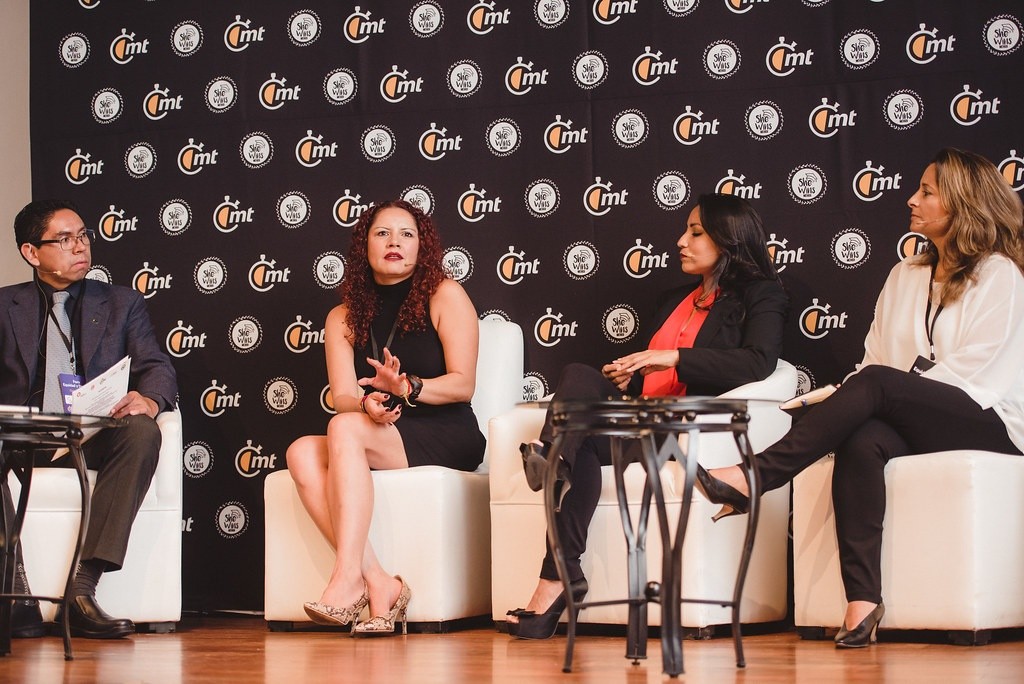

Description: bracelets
[360,394,368,415]
[400,377,412,401]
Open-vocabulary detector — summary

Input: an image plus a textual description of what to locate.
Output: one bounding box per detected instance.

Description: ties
[42,292,77,438]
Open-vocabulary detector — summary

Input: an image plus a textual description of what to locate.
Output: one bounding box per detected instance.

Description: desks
[536,395,761,679]
[0,412,130,661]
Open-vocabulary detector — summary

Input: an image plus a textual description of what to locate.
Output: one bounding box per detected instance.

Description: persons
[693,149,1023,652]
[505,194,780,646]
[2,199,177,643]
[285,199,486,645]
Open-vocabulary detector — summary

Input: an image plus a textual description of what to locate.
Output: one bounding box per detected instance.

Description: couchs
[263,322,524,634]
[0,406,183,636]
[489,358,799,640]
[794,447,1024,648]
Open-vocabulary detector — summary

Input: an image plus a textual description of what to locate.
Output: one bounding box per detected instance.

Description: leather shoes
[52,595,136,638]
[8,603,45,638]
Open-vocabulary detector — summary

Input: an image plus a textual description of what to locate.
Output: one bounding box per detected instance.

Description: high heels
[304,578,370,636]
[506,576,588,639]
[695,463,750,523]
[520,439,573,513]
[355,574,411,635]
[834,601,885,647]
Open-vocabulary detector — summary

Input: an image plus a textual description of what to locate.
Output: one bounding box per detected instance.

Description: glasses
[29,228,95,251]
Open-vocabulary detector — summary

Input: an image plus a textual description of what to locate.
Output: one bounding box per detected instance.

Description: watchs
[404,374,423,404]
